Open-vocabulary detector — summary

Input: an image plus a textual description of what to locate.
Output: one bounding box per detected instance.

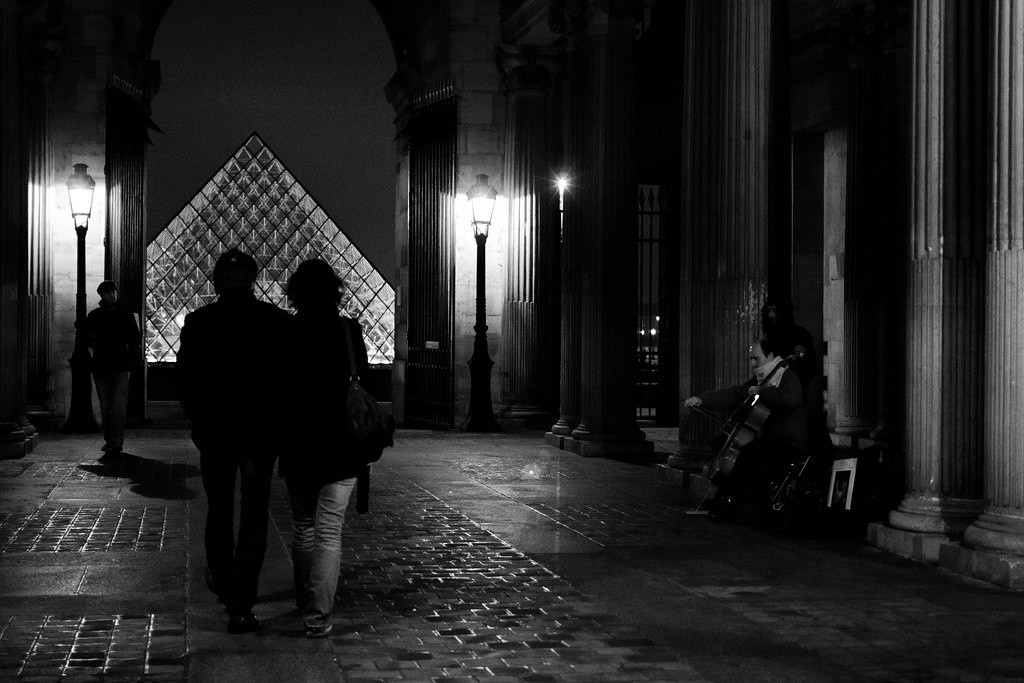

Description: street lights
[460,173,500,437]
[57,162,104,437]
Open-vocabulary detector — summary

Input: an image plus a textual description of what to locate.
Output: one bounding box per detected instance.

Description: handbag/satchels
[343,316,397,463]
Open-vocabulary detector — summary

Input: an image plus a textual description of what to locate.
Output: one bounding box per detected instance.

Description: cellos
[702,345,807,484]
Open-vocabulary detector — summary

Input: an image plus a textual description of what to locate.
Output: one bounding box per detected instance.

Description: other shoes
[307,624,332,637]
[355,486,370,515]
[708,495,738,522]
[227,611,262,634]
[205,566,233,598]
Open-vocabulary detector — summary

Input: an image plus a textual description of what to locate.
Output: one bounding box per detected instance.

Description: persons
[683,295,817,523]
[77,280,142,463]
[175,247,395,639]
